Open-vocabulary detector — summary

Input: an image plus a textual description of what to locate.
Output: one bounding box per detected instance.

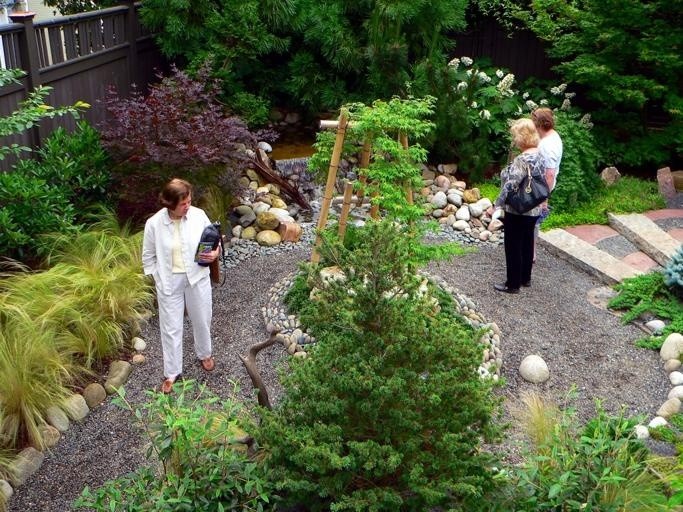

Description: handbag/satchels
[505,157,550,214]
[196,220,228,289]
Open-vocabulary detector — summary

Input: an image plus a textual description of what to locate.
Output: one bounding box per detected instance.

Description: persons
[494,118,547,294]
[142,177,222,394]
[531,108,563,264]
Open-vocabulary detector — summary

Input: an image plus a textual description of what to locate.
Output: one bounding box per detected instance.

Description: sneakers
[161,374,180,394]
[201,358,215,371]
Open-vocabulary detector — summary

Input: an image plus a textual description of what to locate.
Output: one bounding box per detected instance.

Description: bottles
[535,206,551,229]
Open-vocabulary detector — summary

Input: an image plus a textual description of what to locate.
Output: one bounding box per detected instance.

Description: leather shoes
[494,282,520,294]
[522,278,531,287]
